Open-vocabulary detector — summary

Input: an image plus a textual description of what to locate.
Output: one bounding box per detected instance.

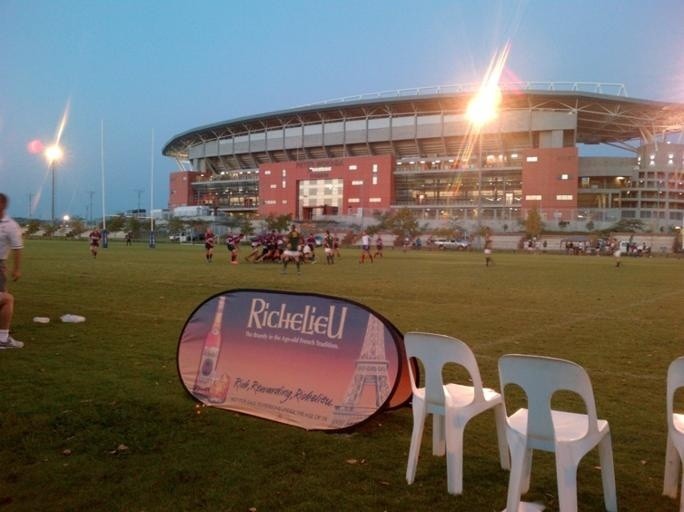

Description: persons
[226,224,383,274]
[526,237,541,254]
[89,227,101,256]
[125,232,132,245]
[0,291,24,350]
[483,232,496,267]
[0,193,24,292]
[205,228,215,261]
[565,238,649,267]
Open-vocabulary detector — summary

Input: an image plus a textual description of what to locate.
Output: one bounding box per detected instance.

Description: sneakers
[0,335,25,350]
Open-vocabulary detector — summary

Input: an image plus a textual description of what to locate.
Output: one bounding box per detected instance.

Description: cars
[167,231,208,243]
[432,237,469,252]
[613,239,643,255]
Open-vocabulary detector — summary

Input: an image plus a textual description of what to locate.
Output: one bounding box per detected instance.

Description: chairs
[403,330,511,497]
[662,356,684,512]
[497,354,618,512]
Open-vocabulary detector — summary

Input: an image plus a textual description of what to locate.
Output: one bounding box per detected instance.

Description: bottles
[59,314,85,324]
[193,295,226,400]
[32,316,50,324]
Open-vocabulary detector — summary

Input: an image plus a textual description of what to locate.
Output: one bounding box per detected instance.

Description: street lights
[470,94,499,250]
[46,144,61,219]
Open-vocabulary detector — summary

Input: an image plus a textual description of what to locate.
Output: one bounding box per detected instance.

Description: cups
[206,372,230,404]
